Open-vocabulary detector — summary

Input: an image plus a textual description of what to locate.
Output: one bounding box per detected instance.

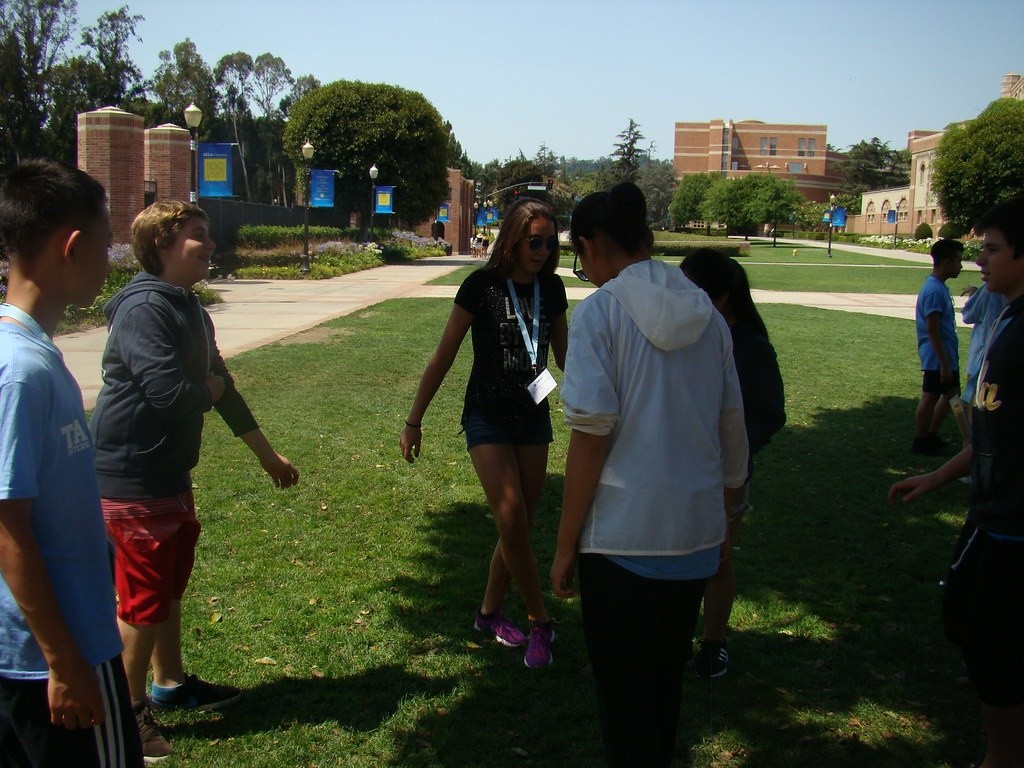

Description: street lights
[483,199,493,236]
[370,164,379,242]
[474,201,478,235]
[301,140,313,276]
[792,211,796,238]
[827,194,836,257]
[894,198,898,247]
[185,100,203,204]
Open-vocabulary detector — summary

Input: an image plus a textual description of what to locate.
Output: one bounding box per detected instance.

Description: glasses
[524,236,559,252]
[573,247,589,282]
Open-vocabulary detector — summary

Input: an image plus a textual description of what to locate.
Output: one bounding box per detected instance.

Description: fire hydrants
[793,250,797,256]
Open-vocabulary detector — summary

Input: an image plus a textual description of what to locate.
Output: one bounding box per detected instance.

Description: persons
[961,282,1009,404]
[889,198,1024,768]
[469,234,489,259]
[680,247,787,680]
[399,198,568,672]
[550,182,749,768]
[90,199,298,762]
[912,239,964,455]
[0,158,144,768]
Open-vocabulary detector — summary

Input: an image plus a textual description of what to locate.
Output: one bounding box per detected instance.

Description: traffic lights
[548,179,553,189]
[515,187,518,198]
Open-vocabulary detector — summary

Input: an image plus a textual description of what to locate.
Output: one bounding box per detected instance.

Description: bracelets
[405,420,421,428]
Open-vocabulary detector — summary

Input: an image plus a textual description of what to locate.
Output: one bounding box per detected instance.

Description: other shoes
[912,437,944,457]
[949,666,984,693]
[927,432,950,448]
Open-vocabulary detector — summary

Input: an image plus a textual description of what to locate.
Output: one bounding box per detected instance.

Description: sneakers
[695,638,730,677]
[133,703,174,763]
[474,607,527,648]
[687,649,695,664]
[151,674,241,712]
[524,620,555,669]
